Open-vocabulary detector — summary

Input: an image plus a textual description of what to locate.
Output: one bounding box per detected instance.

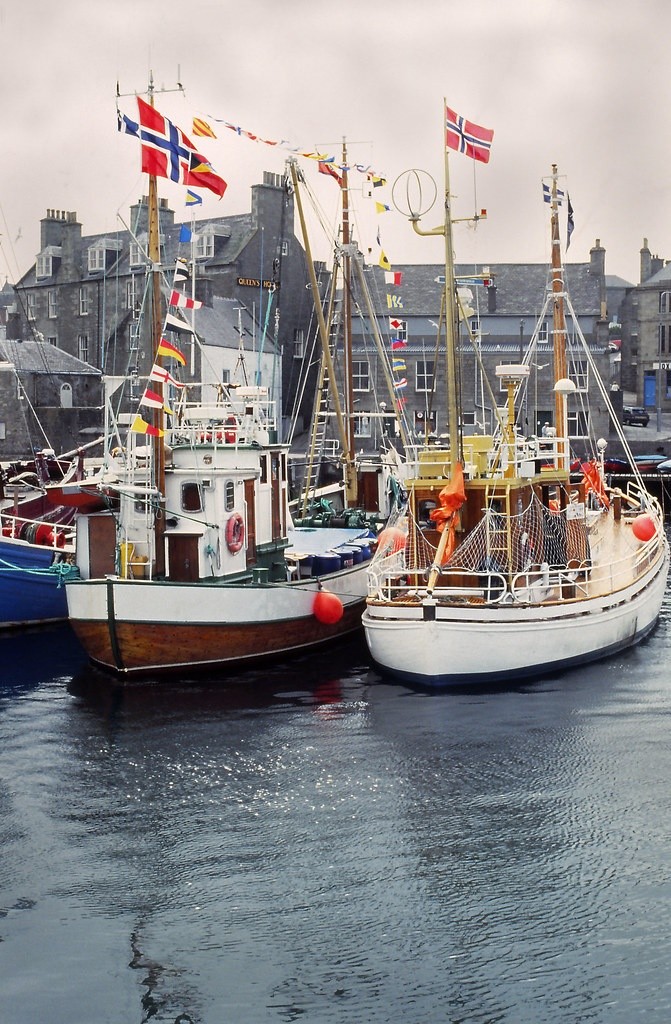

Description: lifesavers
[227,513,245,552]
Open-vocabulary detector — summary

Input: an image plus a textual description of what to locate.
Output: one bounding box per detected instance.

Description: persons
[0,458,27,499]
[541,422,550,437]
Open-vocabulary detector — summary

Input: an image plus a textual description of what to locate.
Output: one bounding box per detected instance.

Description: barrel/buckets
[309,537,378,576]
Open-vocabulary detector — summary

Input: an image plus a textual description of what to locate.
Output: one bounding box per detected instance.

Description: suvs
[622,405,650,428]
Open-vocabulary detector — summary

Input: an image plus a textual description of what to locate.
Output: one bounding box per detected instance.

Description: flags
[217,106,388,189]
[148,363,187,390]
[193,117,216,138]
[371,202,408,411]
[157,339,187,366]
[540,176,575,254]
[137,96,227,198]
[169,290,204,310]
[165,313,194,336]
[446,106,494,163]
[141,388,175,417]
[173,260,189,282]
[186,188,203,206]
[118,109,140,139]
[130,416,169,438]
[179,224,199,243]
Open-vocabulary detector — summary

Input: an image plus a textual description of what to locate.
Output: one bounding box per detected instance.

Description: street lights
[380,402,388,454]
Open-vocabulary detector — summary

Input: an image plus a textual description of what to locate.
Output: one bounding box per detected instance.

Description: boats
[0,454,80,624]
[360,97,669,690]
[61,72,410,680]
